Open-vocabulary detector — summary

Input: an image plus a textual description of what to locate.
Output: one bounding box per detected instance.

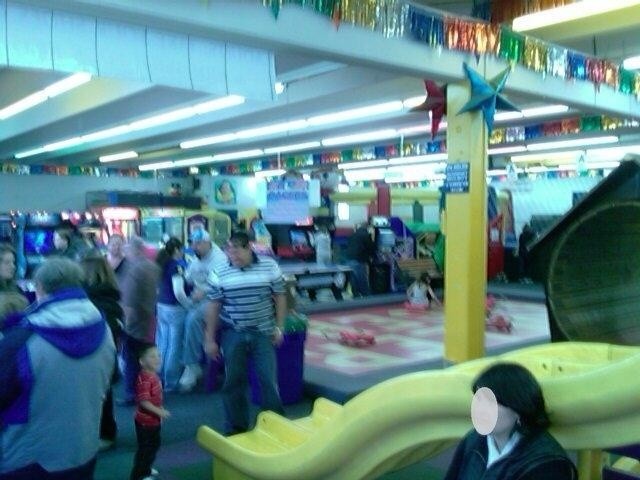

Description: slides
[197,342,640,479]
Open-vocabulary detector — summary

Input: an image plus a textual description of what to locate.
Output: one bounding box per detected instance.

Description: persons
[128,341,170,480]
[1,254,117,480]
[201,231,292,439]
[442,361,580,480]
[0,222,229,403]
